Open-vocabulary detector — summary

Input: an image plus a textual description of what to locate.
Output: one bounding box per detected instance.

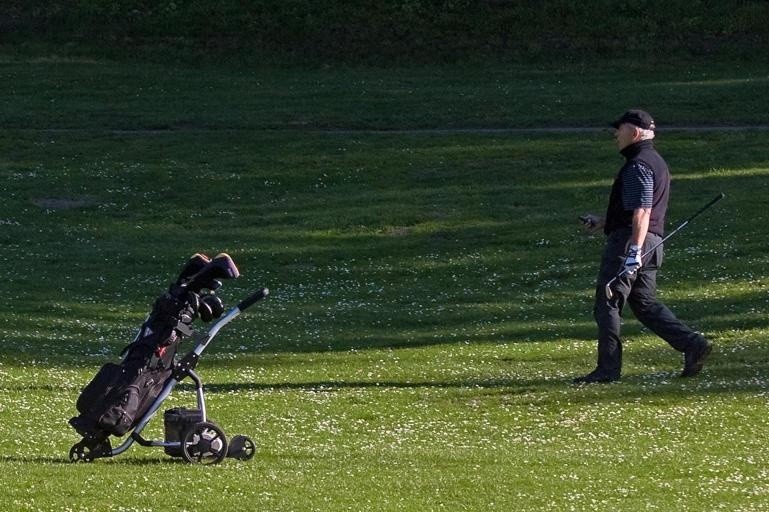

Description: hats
[607,109,657,133]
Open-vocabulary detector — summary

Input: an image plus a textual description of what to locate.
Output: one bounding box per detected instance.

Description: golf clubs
[605,194,725,298]
[176,253,240,323]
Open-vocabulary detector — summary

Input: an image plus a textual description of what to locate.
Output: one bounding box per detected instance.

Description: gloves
[624,244,643,275]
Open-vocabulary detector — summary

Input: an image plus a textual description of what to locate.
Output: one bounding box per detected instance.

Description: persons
[573,109,713,382]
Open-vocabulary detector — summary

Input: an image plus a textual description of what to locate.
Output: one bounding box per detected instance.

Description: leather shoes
[679,334,713,379]
[576,370,623,384]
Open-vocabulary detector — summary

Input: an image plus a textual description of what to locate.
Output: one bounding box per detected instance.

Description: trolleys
[68,279,270,464]
